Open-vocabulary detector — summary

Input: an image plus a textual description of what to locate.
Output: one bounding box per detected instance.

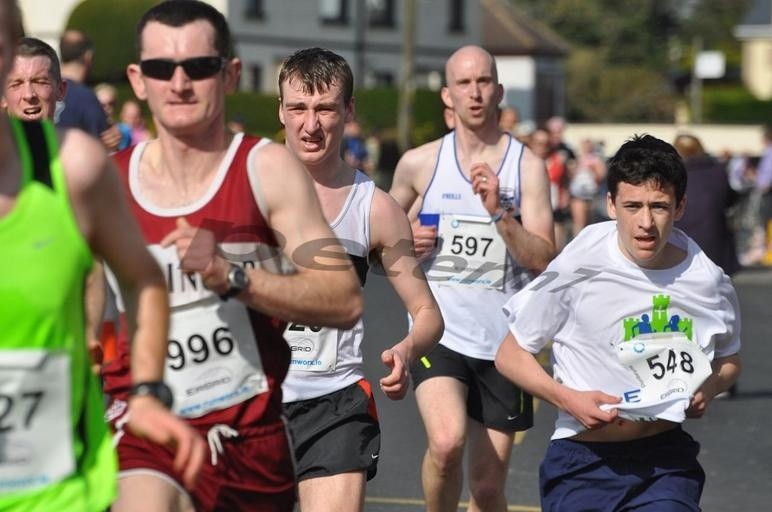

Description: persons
[81,0,365,512]
[2,38,69,121]
[52,28,117,349]
[277,48,446,512]
[382,45,559,511]
[492,132,745,510]
[87,79,772,268]
[0,0,208,512]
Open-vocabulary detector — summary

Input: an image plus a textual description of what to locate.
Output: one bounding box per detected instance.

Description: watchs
[220,261,250,302]
[128,379,174,409]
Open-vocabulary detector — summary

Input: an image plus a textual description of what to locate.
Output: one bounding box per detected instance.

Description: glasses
[139,56,225,80]
